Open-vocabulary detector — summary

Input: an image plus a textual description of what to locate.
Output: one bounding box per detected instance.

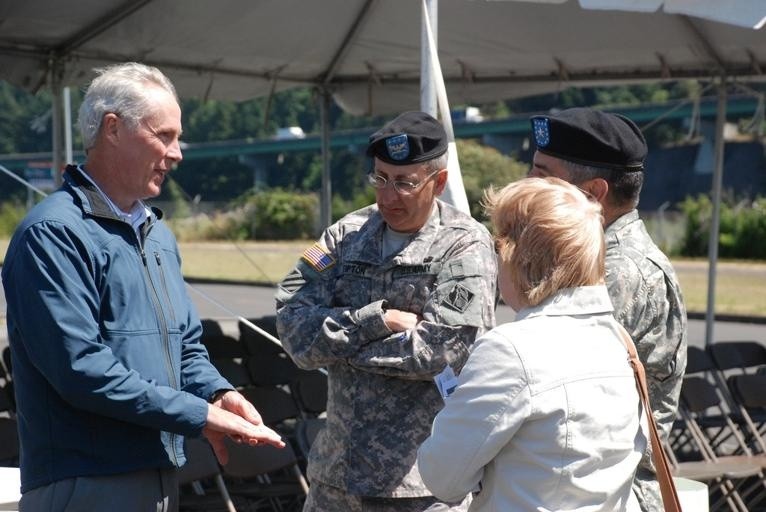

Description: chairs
[662,339,765,512]
[0,314,327,512]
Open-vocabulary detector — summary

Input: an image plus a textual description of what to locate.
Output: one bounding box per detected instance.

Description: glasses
[366,165,437,195]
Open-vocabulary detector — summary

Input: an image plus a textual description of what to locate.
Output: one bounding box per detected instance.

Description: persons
[527,106,689,512]
[416,175,649,511]
[272,109,502,512]
[2,60,287,510]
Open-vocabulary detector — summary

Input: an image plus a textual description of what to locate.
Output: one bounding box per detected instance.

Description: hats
[370,112,448,163]
[530,107,648,170]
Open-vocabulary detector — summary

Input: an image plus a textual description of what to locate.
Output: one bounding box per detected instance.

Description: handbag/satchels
[644,477,709,512]
